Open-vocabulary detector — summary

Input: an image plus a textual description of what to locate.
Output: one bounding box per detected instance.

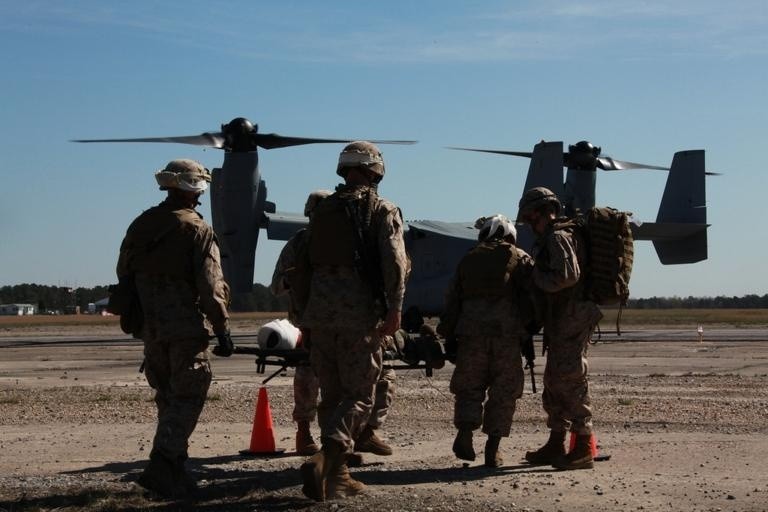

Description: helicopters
[64,112,725,377]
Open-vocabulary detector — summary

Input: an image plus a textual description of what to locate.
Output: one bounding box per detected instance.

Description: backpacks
[305,189,375,266]
[539,206,634,305]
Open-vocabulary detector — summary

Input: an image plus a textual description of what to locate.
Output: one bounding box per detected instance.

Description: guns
[346,199,403,358]
[521,320,538,393]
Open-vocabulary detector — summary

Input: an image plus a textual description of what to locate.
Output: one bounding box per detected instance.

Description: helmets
[155,158,214,193]
[478,214,518,246]
[304,190,333,214]
[337,141,385,183]
[518,187,561,219]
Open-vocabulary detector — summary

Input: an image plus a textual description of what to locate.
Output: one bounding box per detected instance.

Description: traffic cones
[238,383,286,457]
[567,430,611,460]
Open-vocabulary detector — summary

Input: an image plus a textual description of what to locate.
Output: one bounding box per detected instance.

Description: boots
[355,428,392,455]
[553,435,594,469]
[452,427,476,461]
[137,462,193,499]
[485,434,503,466]
[526,430,566,464]
[301,438,345,502]
[296,422,319,456]
[327,456,367,498]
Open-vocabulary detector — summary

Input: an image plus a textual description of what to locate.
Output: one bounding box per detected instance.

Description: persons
[300,142,411,503]
[347,331,396,465]
[443,214,546,469]
[518,187,605,470]
[116,158,238,501]
[270,190,335,454]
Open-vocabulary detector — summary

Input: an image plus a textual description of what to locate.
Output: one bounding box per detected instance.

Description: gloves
[213,336,233,357]
